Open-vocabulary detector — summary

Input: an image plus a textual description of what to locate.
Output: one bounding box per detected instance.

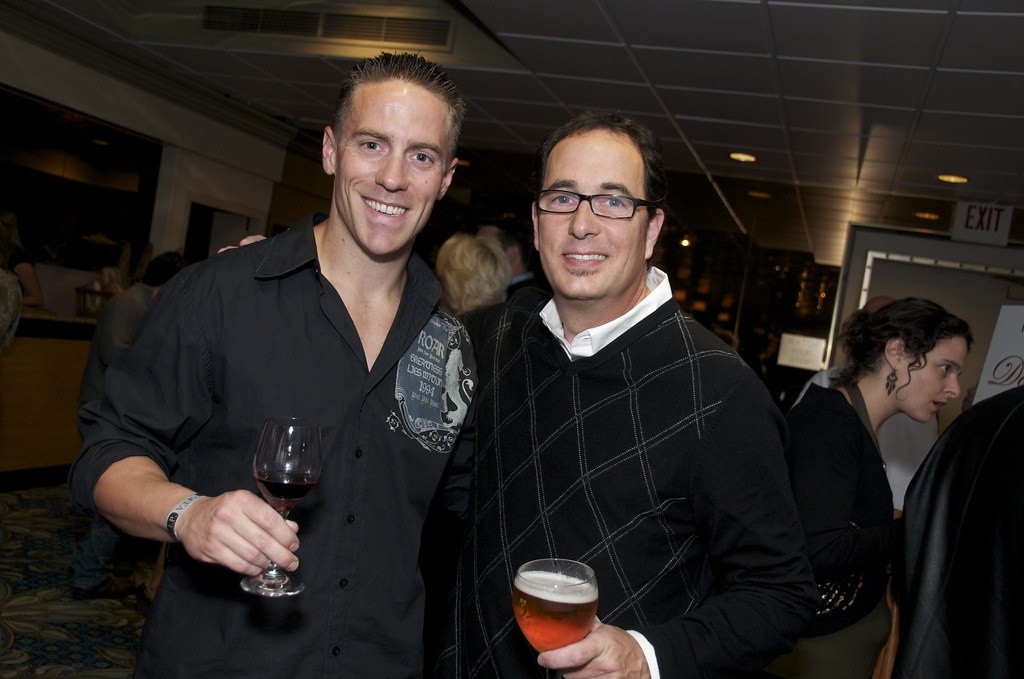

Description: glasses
[535,190,657,219]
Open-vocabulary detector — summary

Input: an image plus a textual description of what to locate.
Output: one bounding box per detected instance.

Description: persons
[67,52,479,679]
[0,210,43,305]
[889,385,1024,679]
[77,251,187,440]
[785,297,974,679]
[437,216,537,317]
[789,295,939,511]
[221,112,818,679]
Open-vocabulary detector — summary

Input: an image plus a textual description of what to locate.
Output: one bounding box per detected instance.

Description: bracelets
[166,492,206,542]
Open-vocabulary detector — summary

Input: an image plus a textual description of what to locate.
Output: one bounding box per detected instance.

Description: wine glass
[240,416,324,597]
[512,558,598,679]
